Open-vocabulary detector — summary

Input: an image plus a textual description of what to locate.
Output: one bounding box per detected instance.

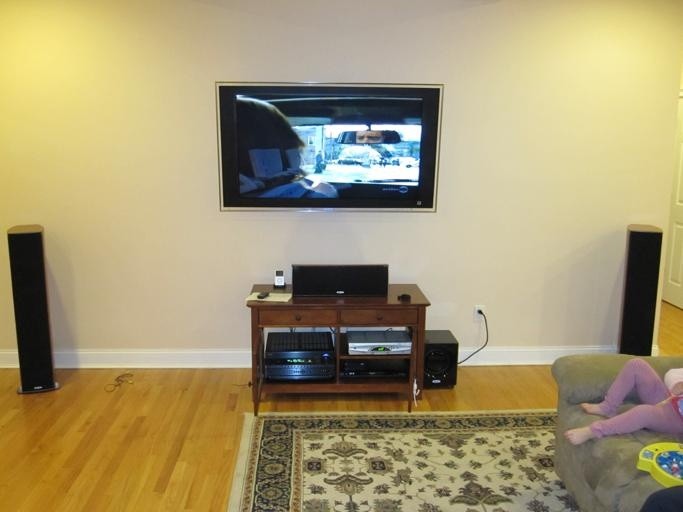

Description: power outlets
[473,305,485,320]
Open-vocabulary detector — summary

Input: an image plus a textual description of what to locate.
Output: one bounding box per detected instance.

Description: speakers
[7,224,60,394]
[425,330,458,386]
[292,264,388,297]
[618,224,663,356]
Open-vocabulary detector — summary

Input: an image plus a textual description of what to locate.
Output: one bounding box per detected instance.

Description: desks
[246,284,431,416]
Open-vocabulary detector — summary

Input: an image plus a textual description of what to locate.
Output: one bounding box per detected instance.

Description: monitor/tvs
[215,82,444,212]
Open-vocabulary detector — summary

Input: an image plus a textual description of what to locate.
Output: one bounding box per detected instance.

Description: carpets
[228,409,577,512]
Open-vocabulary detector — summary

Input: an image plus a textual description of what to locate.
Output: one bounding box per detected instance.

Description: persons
[314,149,322,173]
[563,355,682,448]
[236,96,336,199]
[352,130,385,143]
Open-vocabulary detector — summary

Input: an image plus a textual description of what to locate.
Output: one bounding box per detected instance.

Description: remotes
[258,292,269,299]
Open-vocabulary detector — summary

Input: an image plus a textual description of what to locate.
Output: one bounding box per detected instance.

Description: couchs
[550,354,683,512]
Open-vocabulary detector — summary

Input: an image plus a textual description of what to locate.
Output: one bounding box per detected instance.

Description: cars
[336,145,370,169]
